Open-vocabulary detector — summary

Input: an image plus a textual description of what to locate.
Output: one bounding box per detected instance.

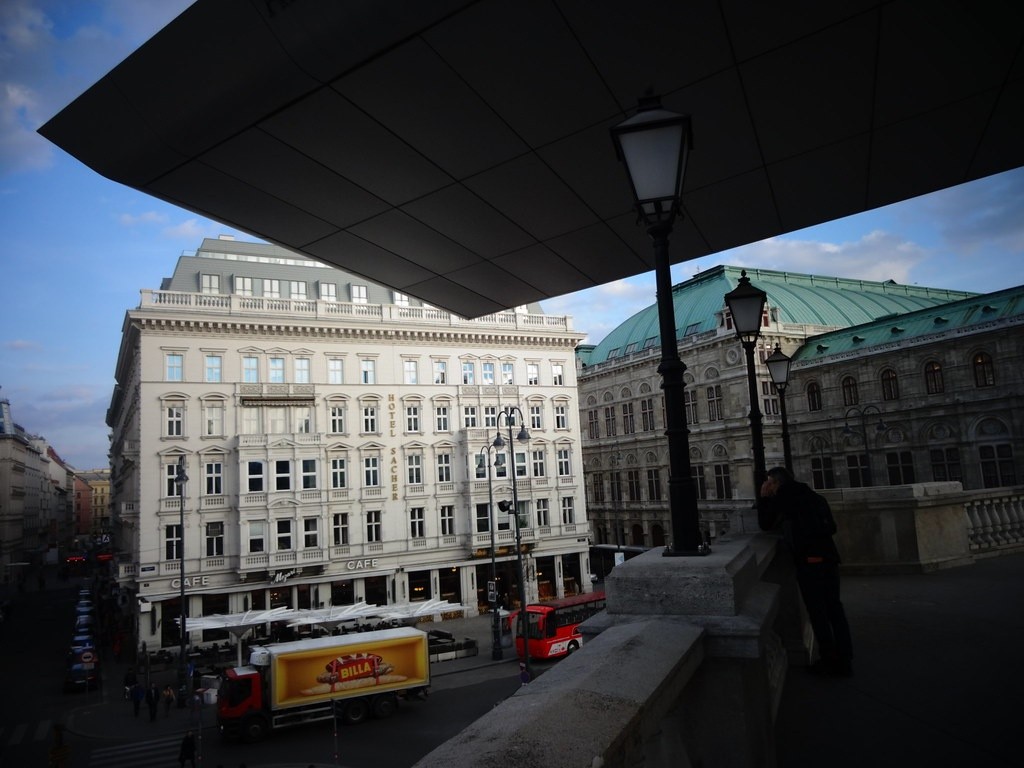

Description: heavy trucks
[214,626,431,744]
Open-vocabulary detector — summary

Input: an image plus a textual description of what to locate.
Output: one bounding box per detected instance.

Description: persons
[115,632,175,724]
[179,730,196,768]
[758,467,858,680]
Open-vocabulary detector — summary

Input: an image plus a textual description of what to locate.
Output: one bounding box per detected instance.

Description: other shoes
[810,653,854,679]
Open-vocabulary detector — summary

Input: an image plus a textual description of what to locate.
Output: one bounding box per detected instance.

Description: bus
[508,590,606,660]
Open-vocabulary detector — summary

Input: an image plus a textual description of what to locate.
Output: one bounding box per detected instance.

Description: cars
[66,588,102,695]
[590,574,598,583]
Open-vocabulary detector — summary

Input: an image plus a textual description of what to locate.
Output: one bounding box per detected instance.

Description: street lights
[173,453,190,686]
[723,269,769,497]
[762,343,795,480]
[492,406,532,680]
[477,443,511,661]
[615,106,714,556]
